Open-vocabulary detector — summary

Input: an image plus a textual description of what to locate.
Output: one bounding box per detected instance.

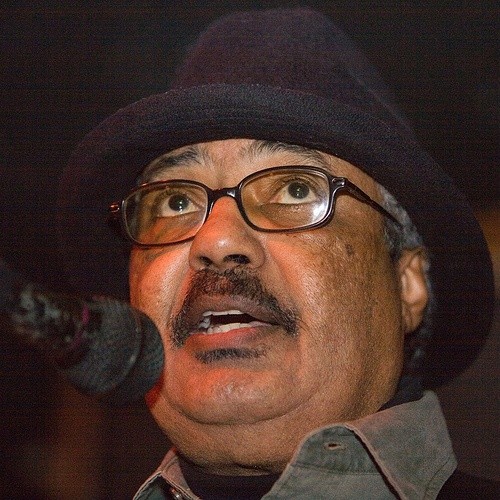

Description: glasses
[109,165,405,249]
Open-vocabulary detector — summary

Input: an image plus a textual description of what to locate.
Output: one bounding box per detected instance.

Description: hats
[57,5,495,391]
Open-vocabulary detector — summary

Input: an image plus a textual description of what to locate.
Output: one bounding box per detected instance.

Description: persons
[54,7,500,500]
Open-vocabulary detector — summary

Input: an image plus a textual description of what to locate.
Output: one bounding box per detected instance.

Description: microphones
[0,259,167,406]
